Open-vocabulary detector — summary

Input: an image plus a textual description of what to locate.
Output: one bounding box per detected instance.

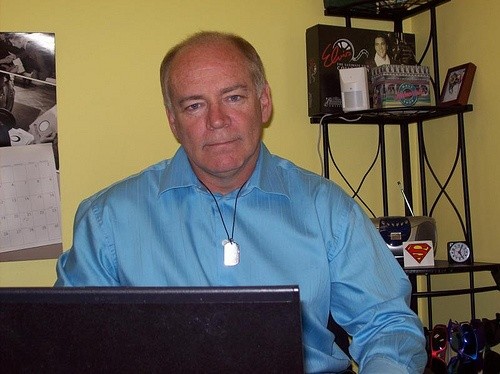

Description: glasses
[430,325,449,374]
[448,319,464,374]
[423,327,429,374]
[461,323,479,374]
[473,318,500,374]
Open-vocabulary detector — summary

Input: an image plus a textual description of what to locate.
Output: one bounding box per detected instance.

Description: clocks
[445,241,473,266]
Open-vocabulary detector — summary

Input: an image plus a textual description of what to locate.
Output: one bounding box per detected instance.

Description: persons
[370,34,400,68]
[52,31,428,374]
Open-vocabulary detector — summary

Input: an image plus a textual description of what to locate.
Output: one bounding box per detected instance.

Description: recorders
[368,180,438,265]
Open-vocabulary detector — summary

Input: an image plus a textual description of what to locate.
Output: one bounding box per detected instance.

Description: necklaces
[198,179,247,267]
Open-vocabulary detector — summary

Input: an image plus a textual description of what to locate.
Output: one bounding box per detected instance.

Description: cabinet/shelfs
[310,0,500,374]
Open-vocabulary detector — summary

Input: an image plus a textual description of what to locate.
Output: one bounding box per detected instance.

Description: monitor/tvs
[0,285,305,374]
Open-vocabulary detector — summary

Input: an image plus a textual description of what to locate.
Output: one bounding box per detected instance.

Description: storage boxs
[305,24,416,116]
